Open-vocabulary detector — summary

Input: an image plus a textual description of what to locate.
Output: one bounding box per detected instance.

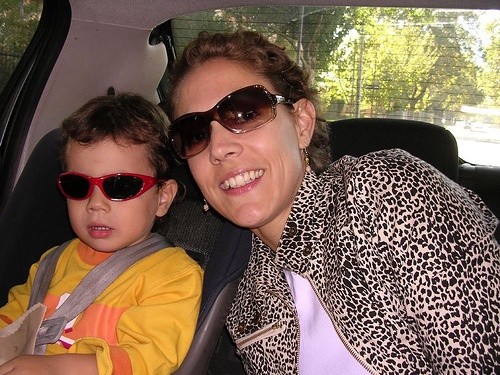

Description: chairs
[0,128,244,374]
[202,117,460,374]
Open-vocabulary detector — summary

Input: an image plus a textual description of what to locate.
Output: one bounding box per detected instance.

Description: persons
[0,89,205,375]
[157,28,500,375]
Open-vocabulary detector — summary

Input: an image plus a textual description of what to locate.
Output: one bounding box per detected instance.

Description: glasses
[167,84,292,163]
[56,172,165,202]
